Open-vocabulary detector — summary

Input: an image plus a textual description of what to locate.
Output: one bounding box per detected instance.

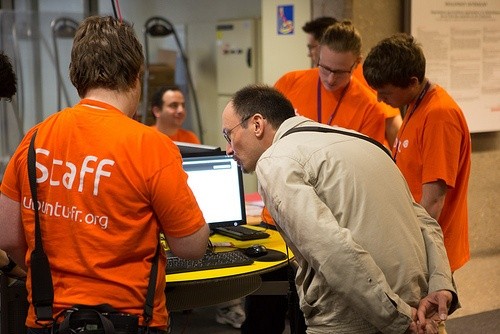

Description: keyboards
[163,249,253,275]
[213,224,270,241]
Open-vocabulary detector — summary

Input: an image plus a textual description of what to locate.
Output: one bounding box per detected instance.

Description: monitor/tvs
[180,151,246,237]
[174,141,223,157]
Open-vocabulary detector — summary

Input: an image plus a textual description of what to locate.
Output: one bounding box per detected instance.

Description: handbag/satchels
[50,303,139,334]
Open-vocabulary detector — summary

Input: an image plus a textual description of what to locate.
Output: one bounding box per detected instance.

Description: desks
[4,223,305,334]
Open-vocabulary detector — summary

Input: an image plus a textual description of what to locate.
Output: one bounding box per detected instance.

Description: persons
[0,54,28,277]
[362,35,472,272]
[0,15,210,334]
[303,16,403,154]
[222,84,461,334]
[274,20,385,145]
[148,83,202,144]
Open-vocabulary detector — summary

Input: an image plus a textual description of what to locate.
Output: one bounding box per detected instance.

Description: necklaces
[78,98,122,113]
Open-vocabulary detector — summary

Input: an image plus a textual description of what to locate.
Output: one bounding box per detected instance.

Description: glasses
[316,56,358,77]
[223,112,266,144]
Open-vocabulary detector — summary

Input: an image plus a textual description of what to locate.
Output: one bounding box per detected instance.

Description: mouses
[244,244,268,257]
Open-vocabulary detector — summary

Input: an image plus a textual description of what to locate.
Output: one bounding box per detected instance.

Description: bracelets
[0,257,17,273]
